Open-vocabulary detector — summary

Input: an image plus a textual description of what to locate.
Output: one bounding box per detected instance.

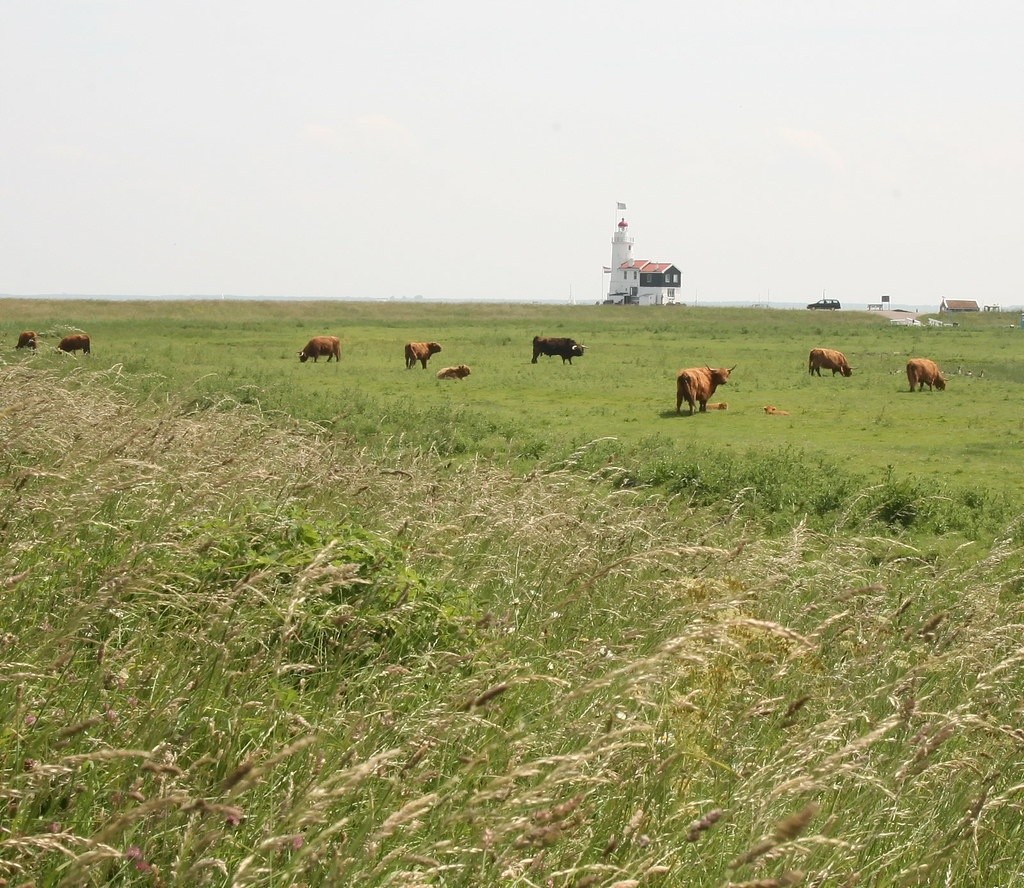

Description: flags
[618,203,626,210]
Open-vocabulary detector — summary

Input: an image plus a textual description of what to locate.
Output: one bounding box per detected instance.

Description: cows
[763,406,790,415]
[906,359,949,393]
[437,364,472,379]
[404,342,442,369]
[676,363,737,414]
[530,336,590,365]
[296,336,342,362]
[808,348,853,377]
[15,331,38,350]
[706,400,729,410]
[57,335,91,355]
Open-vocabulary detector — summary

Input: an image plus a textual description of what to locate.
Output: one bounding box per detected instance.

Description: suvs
[807,299,841,311]
